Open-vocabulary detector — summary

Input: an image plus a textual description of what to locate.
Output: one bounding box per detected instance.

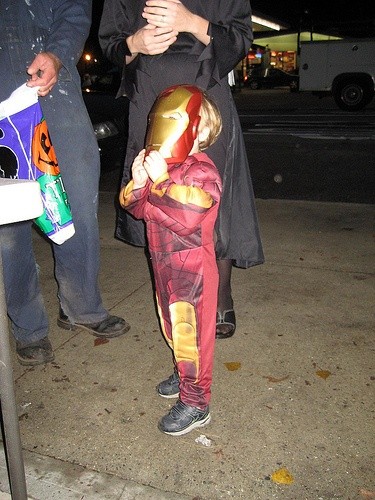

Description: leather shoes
[16,336,55,365]
[57,308,130,338]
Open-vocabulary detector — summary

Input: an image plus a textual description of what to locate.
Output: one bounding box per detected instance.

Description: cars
[244,66,298,90]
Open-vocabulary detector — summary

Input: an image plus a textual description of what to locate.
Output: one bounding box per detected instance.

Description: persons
[118,86,223,437]
[97,2,266,341]
[0,0,131,367]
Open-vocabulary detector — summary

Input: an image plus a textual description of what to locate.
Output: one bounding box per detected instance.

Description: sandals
[216,309,236,338]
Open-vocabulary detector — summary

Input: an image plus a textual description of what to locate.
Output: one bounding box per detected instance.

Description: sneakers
[158,369,180,399]
[157,401,211,436]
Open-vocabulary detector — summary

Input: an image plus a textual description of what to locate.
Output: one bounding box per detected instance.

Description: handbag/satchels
[2,79,78,246]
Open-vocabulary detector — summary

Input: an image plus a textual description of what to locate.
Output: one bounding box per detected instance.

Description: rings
[49,85,53,94]
